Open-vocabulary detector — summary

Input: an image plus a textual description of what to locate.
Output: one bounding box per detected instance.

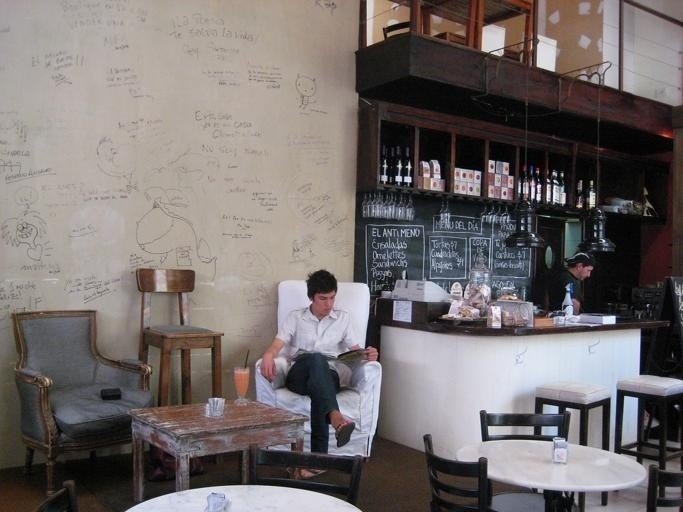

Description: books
[287,348,370,363]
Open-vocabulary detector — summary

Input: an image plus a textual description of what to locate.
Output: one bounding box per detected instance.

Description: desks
[131,399,305,502]
[454,439,646,511]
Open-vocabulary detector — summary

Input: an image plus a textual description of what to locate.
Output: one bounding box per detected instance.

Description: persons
[260,269,379,480]
[548,251,596,316]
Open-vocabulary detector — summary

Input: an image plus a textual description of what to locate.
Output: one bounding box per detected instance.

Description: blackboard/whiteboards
[354,186,535,302]
[669,277,683,338]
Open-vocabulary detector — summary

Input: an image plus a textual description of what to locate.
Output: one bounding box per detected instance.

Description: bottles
[469,253,490,317]
[574,179,596,209]
[517,165,567,209]
[383,271,393,292]
[379,145,412,186]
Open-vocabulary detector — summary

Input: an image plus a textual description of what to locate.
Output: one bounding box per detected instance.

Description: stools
[135,269,228,400]
[535,382,610,511]
[614,370,683,506]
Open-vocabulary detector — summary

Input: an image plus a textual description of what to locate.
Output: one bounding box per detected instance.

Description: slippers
[335,422,355,447]
[300,466,329,479]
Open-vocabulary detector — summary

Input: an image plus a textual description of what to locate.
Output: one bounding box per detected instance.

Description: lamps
[559,61,616,252]
[481,39,548,248]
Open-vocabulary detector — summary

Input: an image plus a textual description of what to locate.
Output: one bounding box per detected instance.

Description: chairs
[477,408,573,501]
[644,460,681,510]
[10,309,151,496]
[420,435,491,512]
[257,282,383,504]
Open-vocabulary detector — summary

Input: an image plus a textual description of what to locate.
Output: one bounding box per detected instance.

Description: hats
[563,251,596,264]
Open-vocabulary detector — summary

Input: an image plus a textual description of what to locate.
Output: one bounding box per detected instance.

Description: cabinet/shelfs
[369,101,667,222]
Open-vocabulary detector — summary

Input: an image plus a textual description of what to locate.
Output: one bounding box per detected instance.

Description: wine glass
[357,193,415,223]
[232,366,251,407]
[433,201,453,227]
[479,204,511,227]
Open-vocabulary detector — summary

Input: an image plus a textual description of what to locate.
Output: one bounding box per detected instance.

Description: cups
[552,436,567,464]
[551,308,566,327]
[207,396,226,417]
[205,493,226,511]
